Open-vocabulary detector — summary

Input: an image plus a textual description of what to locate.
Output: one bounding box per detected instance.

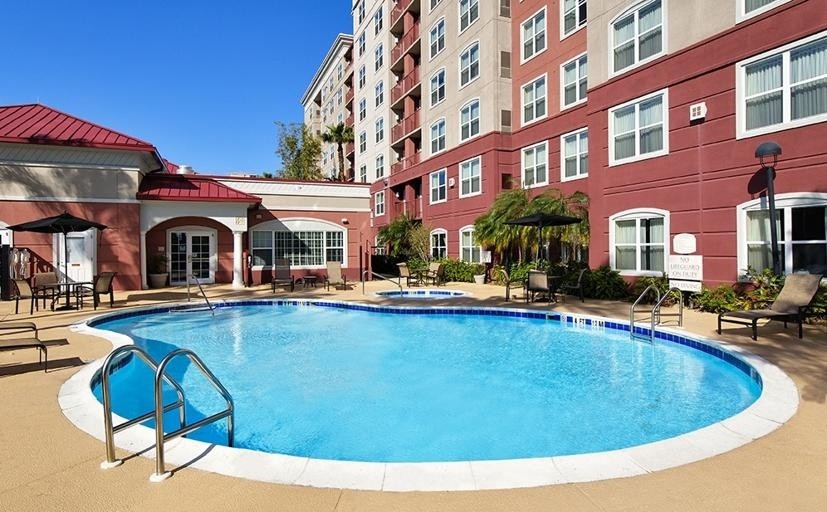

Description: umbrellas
[501,211,584,267]
[3,210,109,283]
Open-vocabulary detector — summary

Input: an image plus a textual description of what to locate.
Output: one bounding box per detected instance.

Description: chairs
[9,272,118,315]
[0,322,38,349]
[499,268,588,305]
[397,262,448,287]
[717,273,823,341]
[323,261,347,291]
[271,259,294,293]
[0,339,47,372]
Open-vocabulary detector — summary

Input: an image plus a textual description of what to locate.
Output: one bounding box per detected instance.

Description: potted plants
[148,253,171,287]
[470,263,488,284]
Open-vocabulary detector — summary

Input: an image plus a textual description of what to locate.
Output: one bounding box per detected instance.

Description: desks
[304,276,317,288]
